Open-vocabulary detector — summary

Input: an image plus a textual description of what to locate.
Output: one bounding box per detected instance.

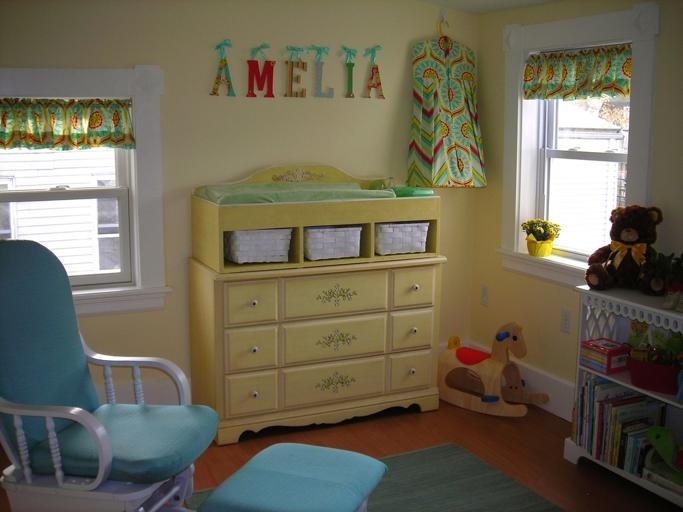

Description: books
[574,336,683,494]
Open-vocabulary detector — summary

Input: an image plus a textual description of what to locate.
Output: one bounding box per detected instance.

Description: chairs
[0,241,219,510]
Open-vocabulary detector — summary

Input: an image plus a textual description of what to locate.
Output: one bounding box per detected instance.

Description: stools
[202,441,388,512]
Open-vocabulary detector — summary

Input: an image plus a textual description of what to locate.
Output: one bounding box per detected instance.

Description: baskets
[304,228,364,261]
[375,221,431,256]
[224,228,294,265]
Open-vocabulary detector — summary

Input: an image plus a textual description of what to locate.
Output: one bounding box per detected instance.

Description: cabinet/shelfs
[563,284,683,509]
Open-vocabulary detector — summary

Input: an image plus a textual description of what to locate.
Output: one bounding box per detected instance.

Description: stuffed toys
[581,204,664,296]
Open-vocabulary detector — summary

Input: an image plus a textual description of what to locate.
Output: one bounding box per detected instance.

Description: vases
[526,240,554,258]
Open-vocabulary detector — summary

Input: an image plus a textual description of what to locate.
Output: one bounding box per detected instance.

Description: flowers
[521,218,561,239]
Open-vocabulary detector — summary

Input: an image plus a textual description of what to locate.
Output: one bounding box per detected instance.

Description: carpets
[186,443,573,511]
